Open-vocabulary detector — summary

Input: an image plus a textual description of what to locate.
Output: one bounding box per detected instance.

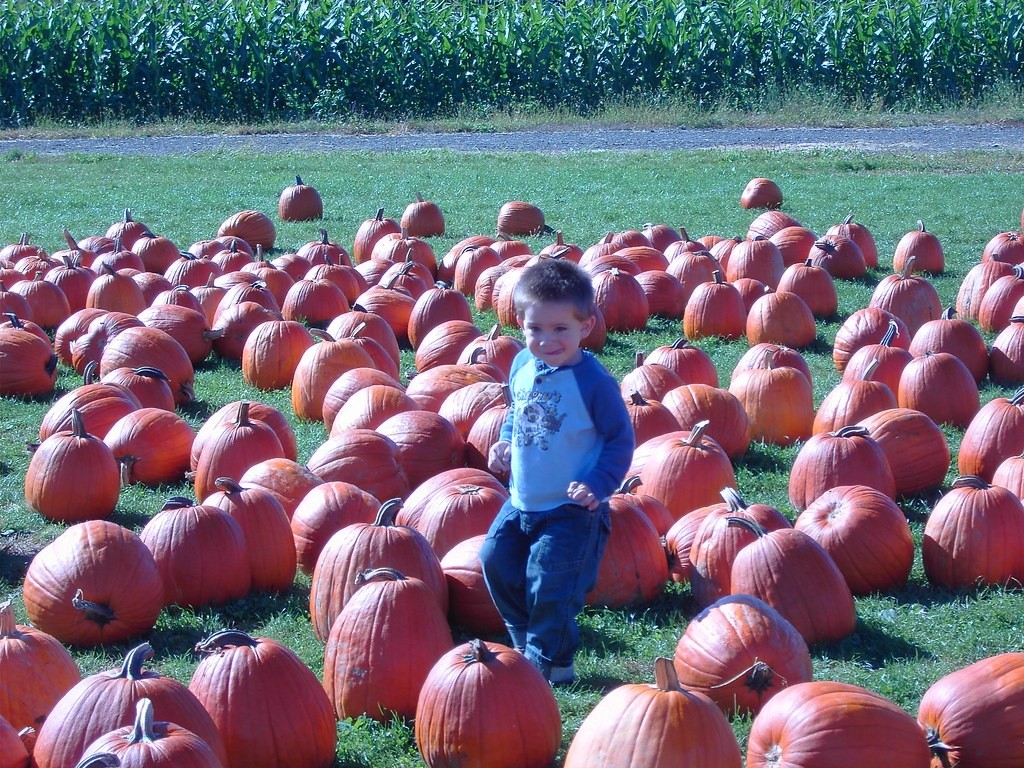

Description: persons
[479,260,636,685]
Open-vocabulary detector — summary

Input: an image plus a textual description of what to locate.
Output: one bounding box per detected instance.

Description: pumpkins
[0,175,1024,768]
